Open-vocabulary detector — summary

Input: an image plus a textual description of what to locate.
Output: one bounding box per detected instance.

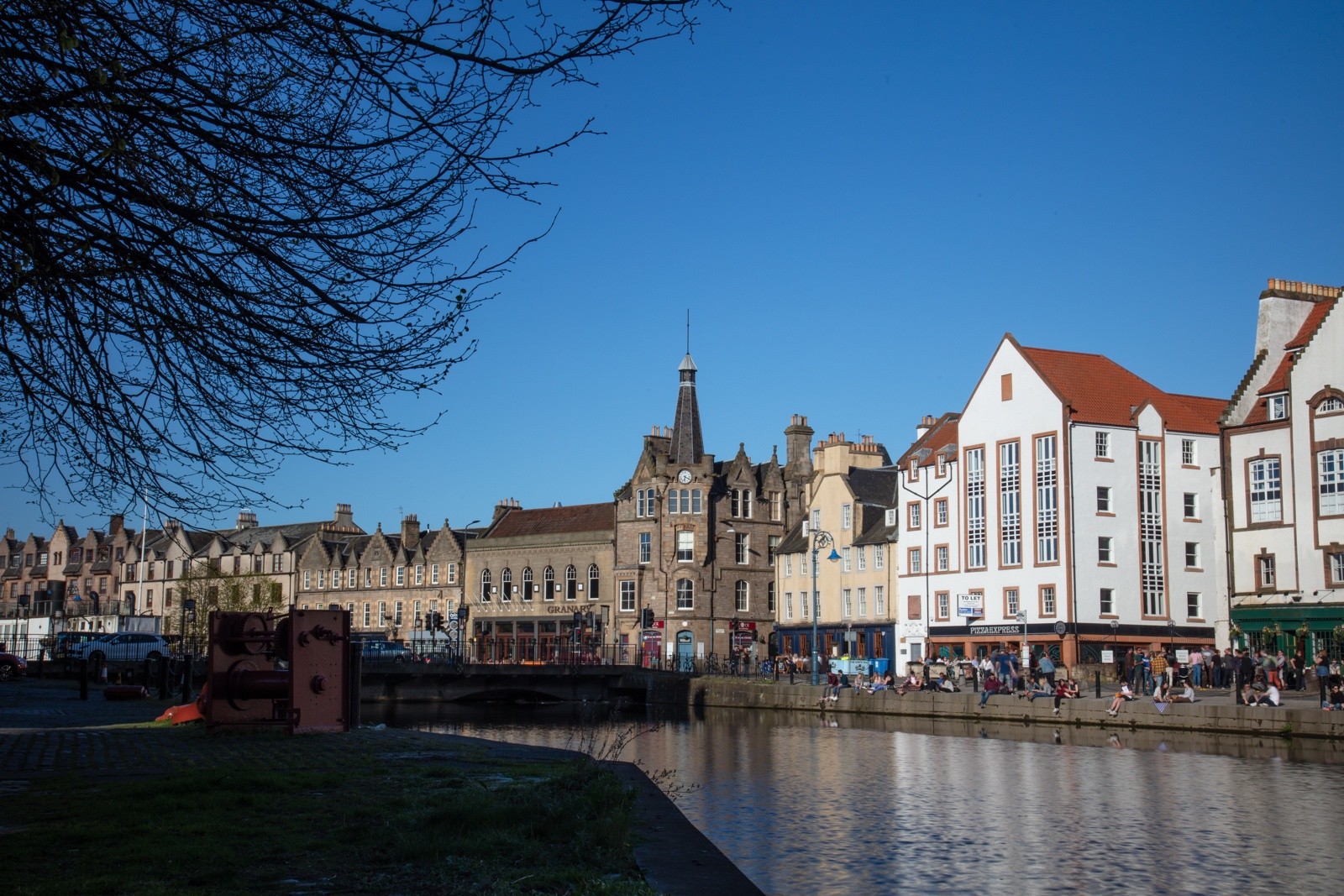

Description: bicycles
[703,651,741,676]
[665,652,682,674]
[683,653,698,676]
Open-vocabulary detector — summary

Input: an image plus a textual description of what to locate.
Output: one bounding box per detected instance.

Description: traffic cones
[98,662,109,685]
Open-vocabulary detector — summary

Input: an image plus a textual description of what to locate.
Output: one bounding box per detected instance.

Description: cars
[416,652,448,664]
[0,652,28,682]
[361,640,412,665]
[68,631,170,661]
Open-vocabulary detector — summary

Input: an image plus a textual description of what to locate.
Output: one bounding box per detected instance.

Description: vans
[55,631,109,661]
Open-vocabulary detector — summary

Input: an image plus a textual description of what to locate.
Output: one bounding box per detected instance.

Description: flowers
[1229,624,1344,644]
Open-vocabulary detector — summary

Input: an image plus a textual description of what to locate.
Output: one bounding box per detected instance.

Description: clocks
[678,469,692,484]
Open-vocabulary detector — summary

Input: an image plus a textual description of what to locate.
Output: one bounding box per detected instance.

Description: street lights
[810,530,843,686]
[138,608,155,634]
[1016,609,1028,645]
[460,519,481,664]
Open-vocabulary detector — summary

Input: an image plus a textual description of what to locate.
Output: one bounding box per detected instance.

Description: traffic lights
[425,613,432,631]
[752,630,758,641]
[435,613,445,631]
[645,608,655,628]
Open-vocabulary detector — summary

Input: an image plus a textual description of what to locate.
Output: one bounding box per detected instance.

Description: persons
[743,646,750,677]
[829,669,848,701]
[867,671,894,694]
[1315,649,1344,710]
[1124,646,1307,707]
[895,646,1080,712]
[784,657,796,684]
[730,645,740,677]
[818,669,838,701]
[853,673,864,696]
[1104,676,1133,715]
[869,671,881,690]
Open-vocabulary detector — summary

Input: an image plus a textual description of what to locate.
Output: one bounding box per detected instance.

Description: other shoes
[1054,708,1059,713]
[1250,702,1256,706]
[1323,707,1330,711]
[820,696,838,701]
[1259,703,1269,707]
[1109,711,1117,715]
[869,687,875,690]
[867,690,873,694]
[862,686,867,689]
[1105,709,1112,712]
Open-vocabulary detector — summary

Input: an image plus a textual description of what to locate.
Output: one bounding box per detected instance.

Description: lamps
[1230,592,1252,598]
[1313,589,1335,595]
[1258,590,1278,597]
[1284,590,1305,595]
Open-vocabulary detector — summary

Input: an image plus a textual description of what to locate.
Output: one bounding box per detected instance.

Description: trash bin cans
[870,658,890,677]
